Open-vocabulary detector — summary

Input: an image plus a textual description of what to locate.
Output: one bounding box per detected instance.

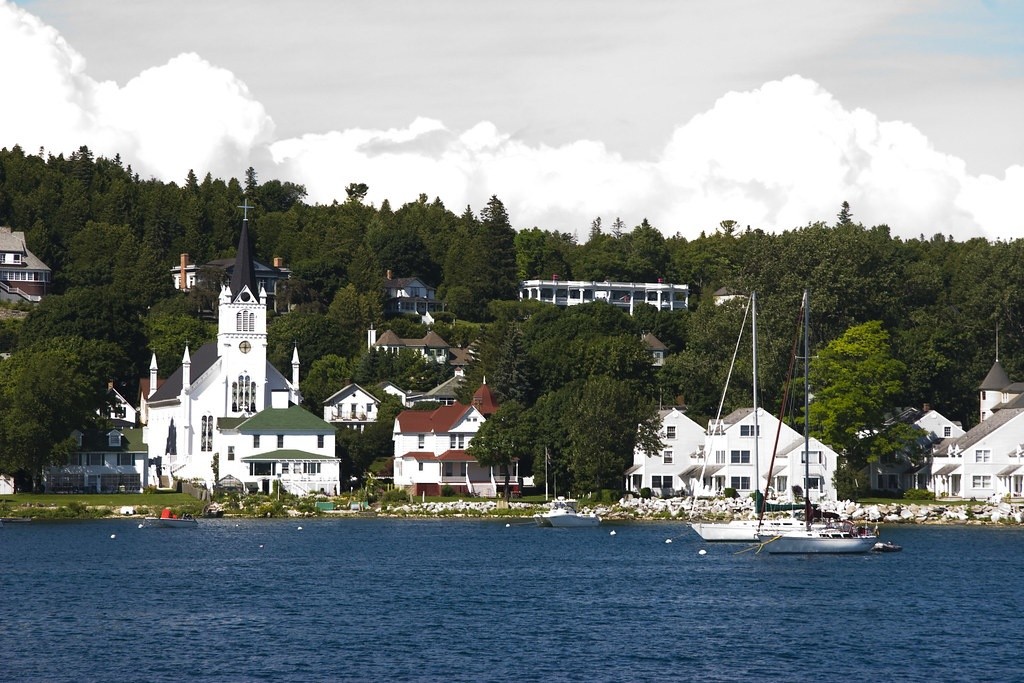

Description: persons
[804,498,814,527]
[334,485,338,496]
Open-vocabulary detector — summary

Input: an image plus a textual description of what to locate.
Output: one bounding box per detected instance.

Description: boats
[874,542,902,552]
[139,514,196,528]
[533,492,602,527]
[202,504,223,517]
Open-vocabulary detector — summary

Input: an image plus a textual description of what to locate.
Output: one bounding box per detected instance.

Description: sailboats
[686,290,878,554]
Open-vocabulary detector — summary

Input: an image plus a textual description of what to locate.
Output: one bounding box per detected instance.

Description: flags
[546,452,551,465]
